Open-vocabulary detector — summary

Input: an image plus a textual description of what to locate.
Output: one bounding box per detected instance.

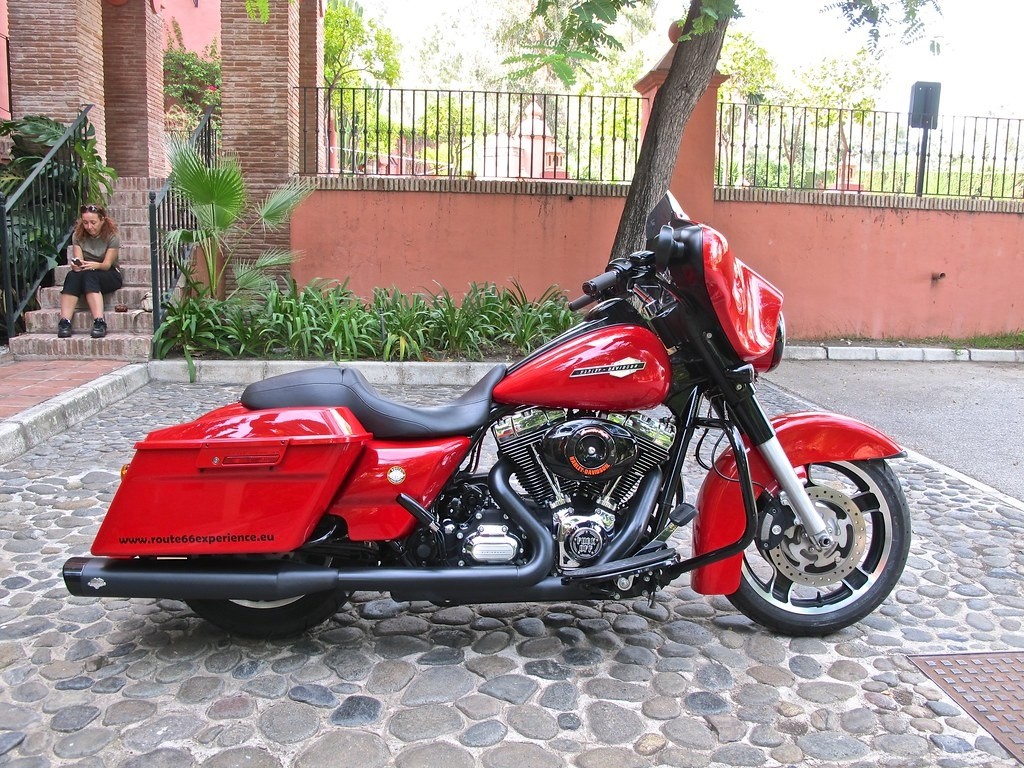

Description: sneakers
[58,318,72,337]
[91,318,107,338]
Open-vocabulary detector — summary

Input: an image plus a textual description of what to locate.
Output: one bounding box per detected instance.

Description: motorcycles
[62,192,912,640]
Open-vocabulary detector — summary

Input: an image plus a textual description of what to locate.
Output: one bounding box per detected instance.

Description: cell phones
[72,258,82,267]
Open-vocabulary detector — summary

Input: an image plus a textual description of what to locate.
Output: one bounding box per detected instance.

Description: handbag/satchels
[140,291,154,312]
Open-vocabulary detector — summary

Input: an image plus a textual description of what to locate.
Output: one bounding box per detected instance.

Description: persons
[58,204,123,338]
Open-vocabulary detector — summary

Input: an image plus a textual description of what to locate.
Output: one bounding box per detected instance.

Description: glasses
[80,205,98,213]
